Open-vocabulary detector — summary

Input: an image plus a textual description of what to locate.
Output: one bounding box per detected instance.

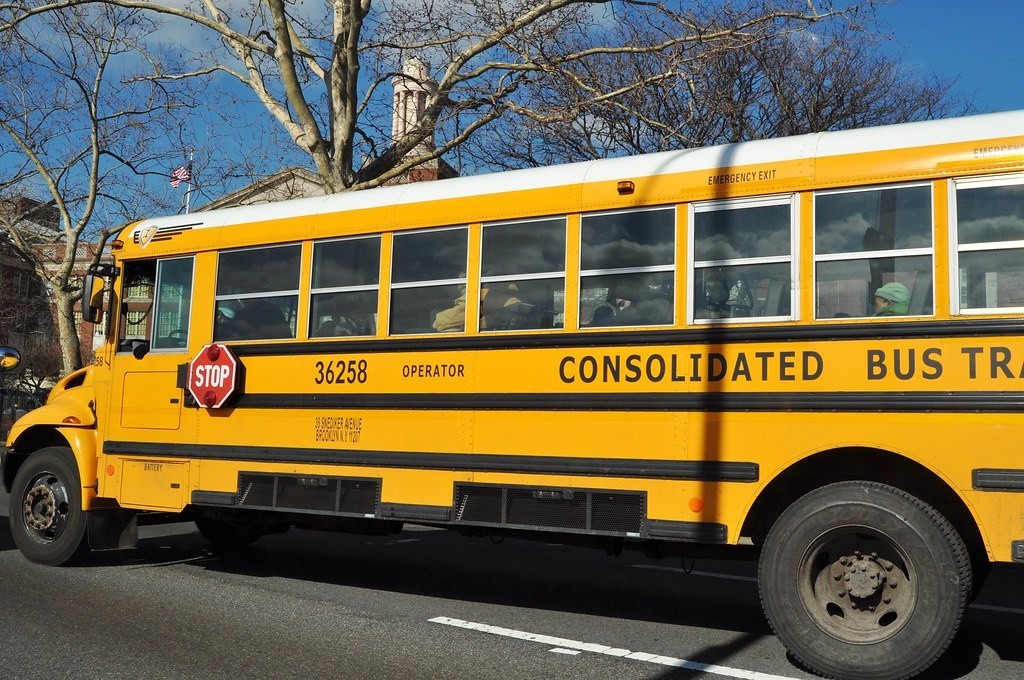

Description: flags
[170,161,192,187]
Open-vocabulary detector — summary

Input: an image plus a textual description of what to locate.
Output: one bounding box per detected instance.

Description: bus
[0,112,1023,678]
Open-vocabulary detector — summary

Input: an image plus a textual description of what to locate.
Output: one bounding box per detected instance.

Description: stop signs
[189,344,235,409]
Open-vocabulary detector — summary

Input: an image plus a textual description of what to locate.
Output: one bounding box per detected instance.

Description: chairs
[906,270,932,315]
[763,275,786,315]
[251,301,292,338]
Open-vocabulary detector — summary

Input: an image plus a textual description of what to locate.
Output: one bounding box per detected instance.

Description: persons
[312,305,368,337]
[433,269,519,332]
[584,278,660,327]
[217,290,293,339]
[872,283,911,317]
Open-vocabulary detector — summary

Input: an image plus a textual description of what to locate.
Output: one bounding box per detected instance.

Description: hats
[874,282,911,303]
